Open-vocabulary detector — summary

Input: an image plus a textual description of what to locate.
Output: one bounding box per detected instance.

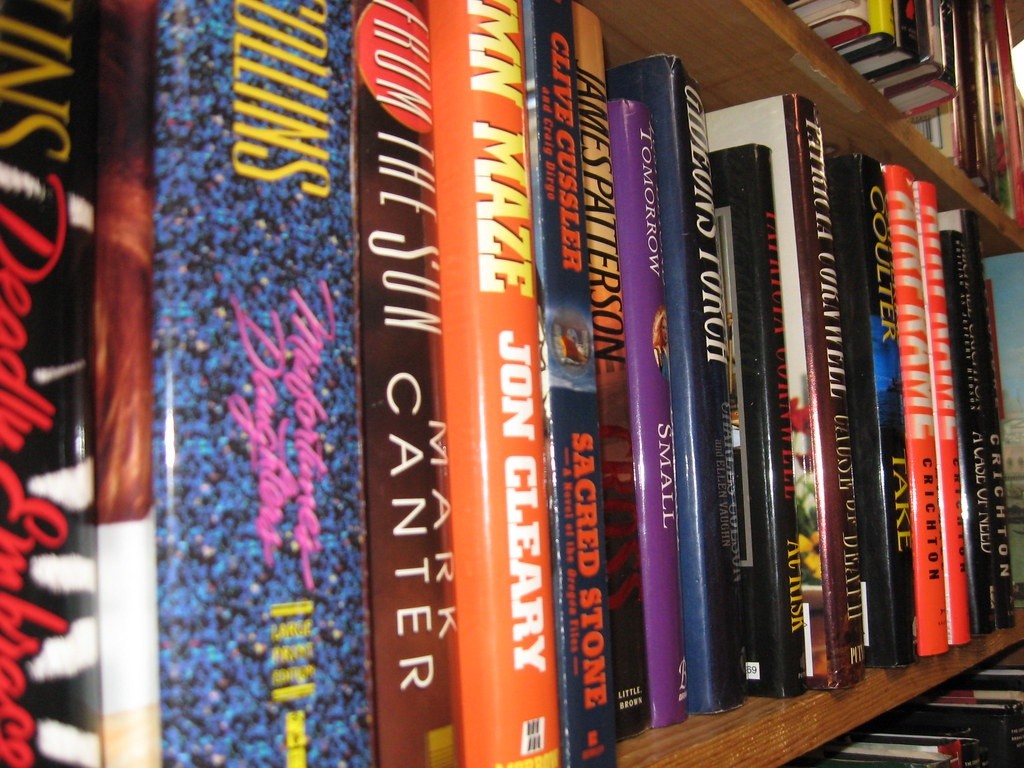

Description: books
[0,0,1024,768]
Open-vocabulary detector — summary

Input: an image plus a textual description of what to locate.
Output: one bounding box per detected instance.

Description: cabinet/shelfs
[595,0,1024,768]
